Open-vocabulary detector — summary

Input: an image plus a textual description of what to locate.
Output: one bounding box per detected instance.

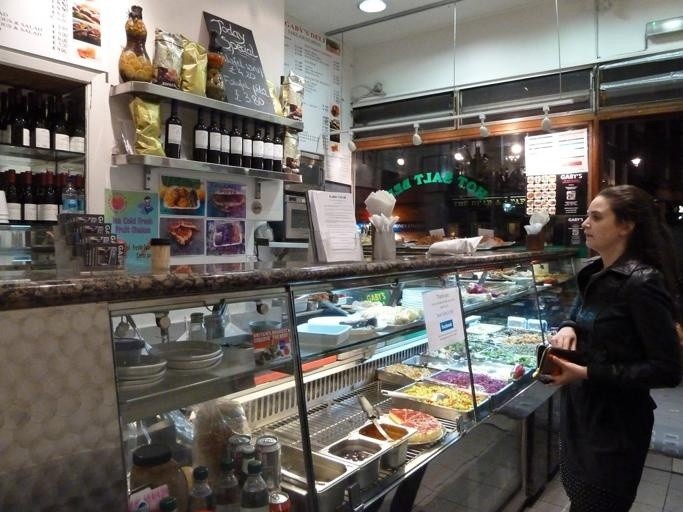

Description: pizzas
[388,407,444,443]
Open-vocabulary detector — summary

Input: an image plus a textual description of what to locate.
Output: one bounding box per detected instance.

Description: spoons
[379,387,449,404]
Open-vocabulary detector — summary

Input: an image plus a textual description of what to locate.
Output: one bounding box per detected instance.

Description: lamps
[478,114,491,139]
[413,122,422,146]
[540,105,552,131]
[347,130,359,153]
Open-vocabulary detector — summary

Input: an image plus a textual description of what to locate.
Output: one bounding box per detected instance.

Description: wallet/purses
[533,344,590,383]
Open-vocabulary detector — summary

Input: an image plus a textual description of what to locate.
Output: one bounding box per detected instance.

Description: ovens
[277,185,324,242]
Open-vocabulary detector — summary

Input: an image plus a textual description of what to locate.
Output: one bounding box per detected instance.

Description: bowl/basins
[293,322,352,348]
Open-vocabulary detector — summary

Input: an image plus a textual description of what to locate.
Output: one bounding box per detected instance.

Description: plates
[162,186,199,210]
[309,316,373,334]
[356,303,416,328]
[150,339,225,375]
[213,224,242,247]
[116,354,168,392]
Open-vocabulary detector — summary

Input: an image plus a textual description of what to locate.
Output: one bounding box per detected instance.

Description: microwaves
[285,150,324,193]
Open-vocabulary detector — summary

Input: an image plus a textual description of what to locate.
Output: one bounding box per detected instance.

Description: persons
[532,183,683,512]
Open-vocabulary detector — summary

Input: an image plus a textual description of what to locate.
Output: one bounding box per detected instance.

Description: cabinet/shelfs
[112,246,578,511]
[0,44,110,283]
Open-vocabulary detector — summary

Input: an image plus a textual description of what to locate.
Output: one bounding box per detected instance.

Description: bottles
[231,116,242,166]
[160,496,178,512]
[190,312,209,343]
[240,460,272,512]
[208,110,221,163]
[252,121,264,169]
[192,107,209,162]
[189,468,216,512]
[1,164,86,227]
[242,117,253,168]
[272,128,283,171]
[263,126,273,170]
[214,458,238,512]
[165,99,182,159]
[240,445,256,483]
[0,86,83,147]
[220,112,231,165]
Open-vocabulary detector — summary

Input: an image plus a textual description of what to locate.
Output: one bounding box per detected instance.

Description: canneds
[234,442,257,462]
[129,445,188,512]
[227,435,251,458]
[256,435,284,487]
[270,490,290,512]
